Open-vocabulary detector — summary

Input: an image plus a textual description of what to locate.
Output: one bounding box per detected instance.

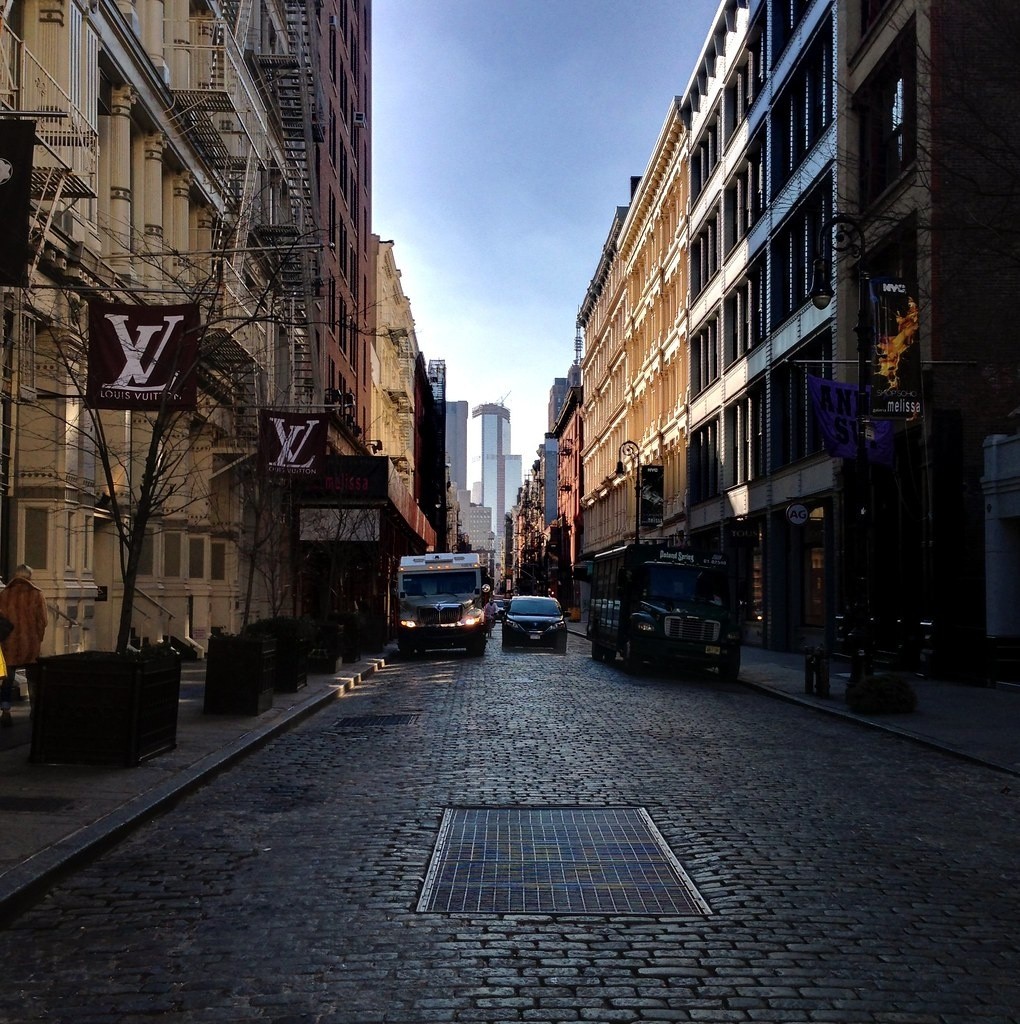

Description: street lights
[807,214,882,678]
[614,441,642,544]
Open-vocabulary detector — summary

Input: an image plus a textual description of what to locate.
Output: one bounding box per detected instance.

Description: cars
[493,595,568,654]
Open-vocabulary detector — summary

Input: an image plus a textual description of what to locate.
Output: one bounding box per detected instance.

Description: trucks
[585,542,742,685]
[395,552,492,663]
